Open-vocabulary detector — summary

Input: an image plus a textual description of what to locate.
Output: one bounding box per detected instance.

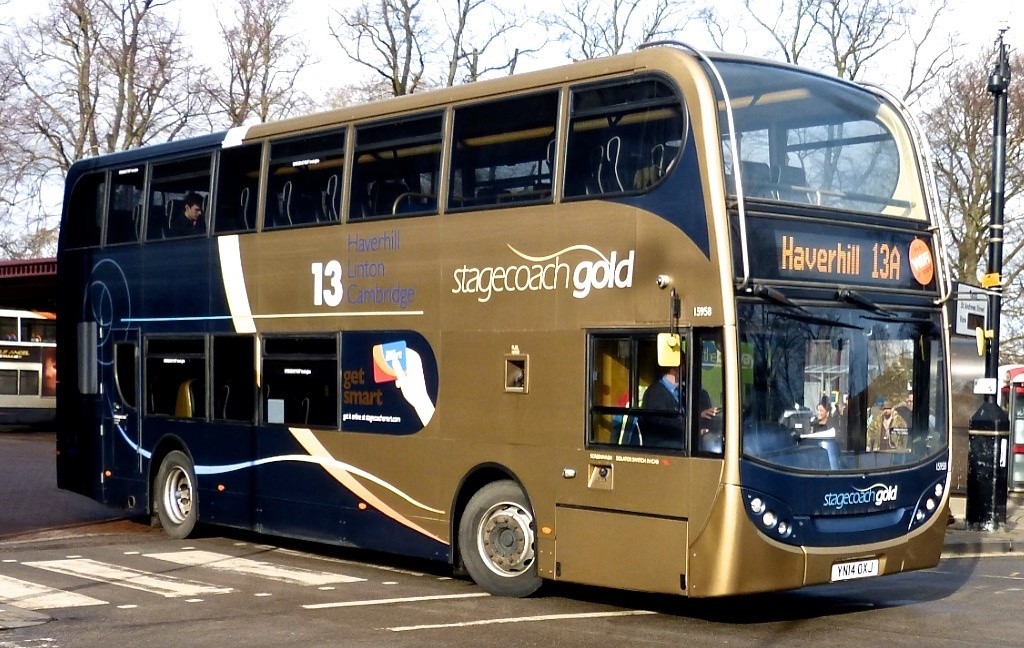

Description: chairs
[624,401,643,446]
[151,379,337,425]
[131,135,817,242]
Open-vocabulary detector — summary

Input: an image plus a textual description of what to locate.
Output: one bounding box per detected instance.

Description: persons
[811,401,847,450]
[641,351,717,449]
[866,402,908,451]
[168,192,205,237]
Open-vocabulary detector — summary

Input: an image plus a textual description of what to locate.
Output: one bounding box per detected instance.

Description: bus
[49,37,995,607]
[998,363,1024,494]
[0,308,58,432]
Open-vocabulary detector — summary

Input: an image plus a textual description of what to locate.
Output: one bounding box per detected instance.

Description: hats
[882,401,892,409]
